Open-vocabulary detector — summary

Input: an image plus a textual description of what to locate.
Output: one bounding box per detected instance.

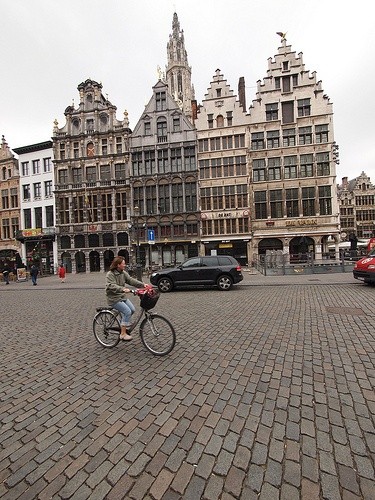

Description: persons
[58,264,66,283]
[2,263,11,284]
[106,256,149,341]
[30,265,39,286]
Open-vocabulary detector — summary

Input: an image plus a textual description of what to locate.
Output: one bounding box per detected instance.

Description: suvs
[149,256,244,293]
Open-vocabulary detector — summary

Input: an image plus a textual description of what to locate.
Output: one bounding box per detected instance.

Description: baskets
[140,289,160,309]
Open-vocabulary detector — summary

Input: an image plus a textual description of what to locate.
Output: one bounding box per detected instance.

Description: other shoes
[33,284,37,286]
[6,283,9,285]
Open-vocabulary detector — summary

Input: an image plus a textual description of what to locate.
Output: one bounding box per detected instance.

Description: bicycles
[92,286,177,357]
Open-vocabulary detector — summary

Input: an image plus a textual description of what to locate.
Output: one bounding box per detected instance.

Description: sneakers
[120,334,133,341]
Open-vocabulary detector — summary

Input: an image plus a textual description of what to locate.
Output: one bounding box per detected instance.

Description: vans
[352,246,375,283]
